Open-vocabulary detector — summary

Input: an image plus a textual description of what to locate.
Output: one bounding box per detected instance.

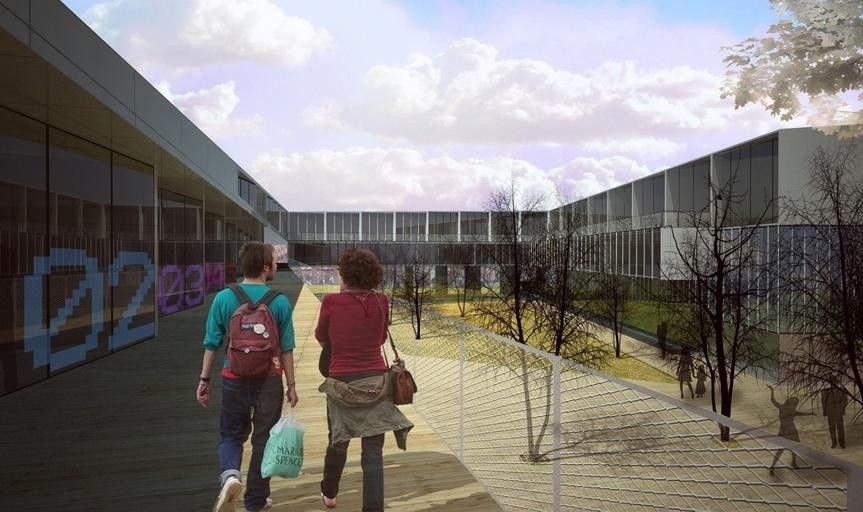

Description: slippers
[320,491,337,509]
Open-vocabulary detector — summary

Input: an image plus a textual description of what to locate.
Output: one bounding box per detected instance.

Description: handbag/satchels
[260,405,304,479]
[389,360,417,406]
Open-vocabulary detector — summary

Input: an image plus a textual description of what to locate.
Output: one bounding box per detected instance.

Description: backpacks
[224,284,284,378]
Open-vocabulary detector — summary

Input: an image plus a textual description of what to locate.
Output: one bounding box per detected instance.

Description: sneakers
[257,498,273,512]
[213,476,242,512]
